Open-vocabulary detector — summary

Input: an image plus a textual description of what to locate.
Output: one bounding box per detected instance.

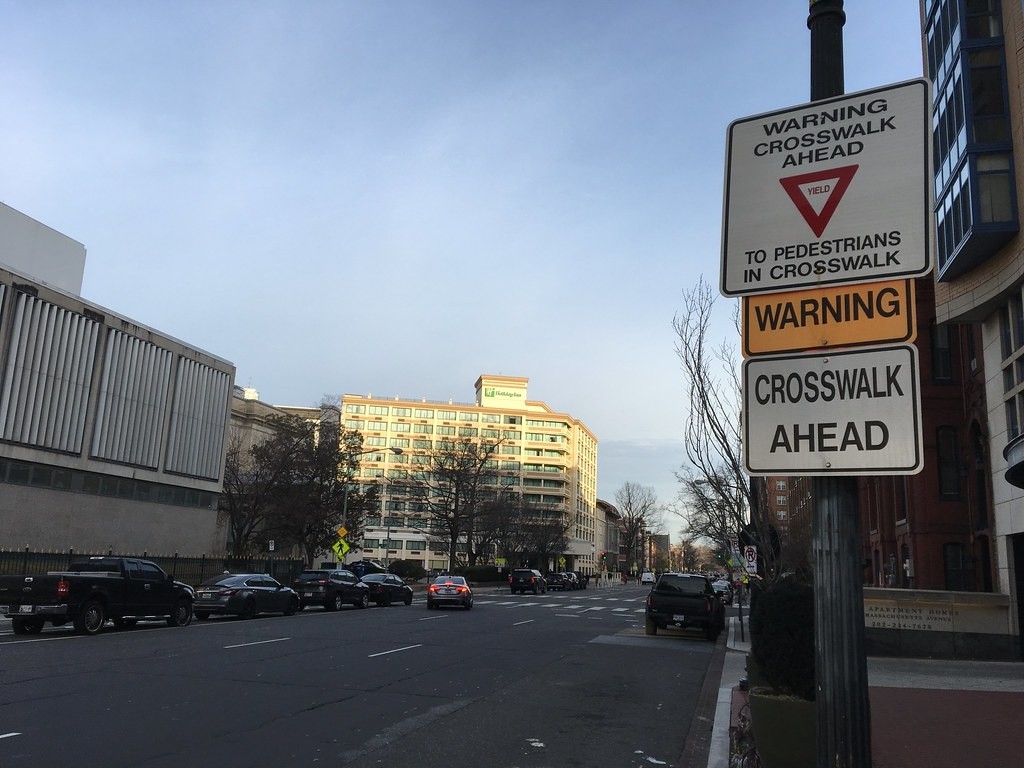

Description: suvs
[646,573,725,641]
[291,568,371,611]
[510,569,547,595]
[547,573,572,592]
[573,571,588,590]
[641,573,656,585]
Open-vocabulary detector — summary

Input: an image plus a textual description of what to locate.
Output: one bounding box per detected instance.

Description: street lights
[342,447,403,529]
[695,479,745,532]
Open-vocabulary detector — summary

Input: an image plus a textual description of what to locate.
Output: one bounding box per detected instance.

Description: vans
[565,572,579,590]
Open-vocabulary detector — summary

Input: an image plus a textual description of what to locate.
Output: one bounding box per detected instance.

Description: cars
[360,572,413,607]
[192,574,300,620]
[712,578,732,605]
[347,561,387,578]
[427,576,473,610]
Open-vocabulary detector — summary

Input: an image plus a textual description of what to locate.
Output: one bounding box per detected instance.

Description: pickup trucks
[1,557,195,636]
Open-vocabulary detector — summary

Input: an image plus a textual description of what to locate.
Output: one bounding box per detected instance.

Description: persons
[622,569,711,585]
[549,569,552,574]
[585,573,590,587]
[594,573,601,586]
